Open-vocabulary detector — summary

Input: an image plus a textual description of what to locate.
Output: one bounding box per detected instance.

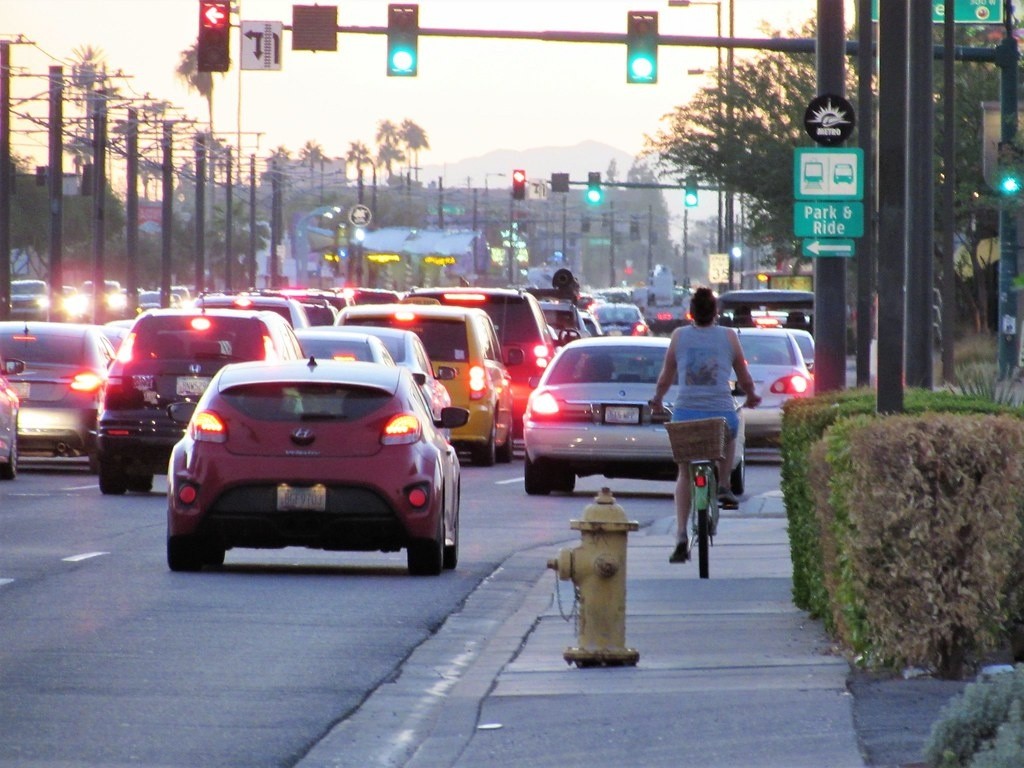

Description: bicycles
[658,400,752,579]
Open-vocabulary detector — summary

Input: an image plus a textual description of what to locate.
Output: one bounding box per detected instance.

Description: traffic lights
[587,172,602,204]
[388,3,419,77]
[684,174,699,206]
[198,0,231,73]
[511,169,525,200]
[627,10,658,83]
[999,174,1018,200]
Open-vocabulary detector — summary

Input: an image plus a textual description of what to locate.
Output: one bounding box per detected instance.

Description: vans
[718,291,815,337]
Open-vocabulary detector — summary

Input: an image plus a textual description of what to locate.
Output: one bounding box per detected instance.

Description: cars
[734,329,814,447]
[60,280,717,464]
[165,355,471,577]
[0,322,117,475]
[522,334,746,495]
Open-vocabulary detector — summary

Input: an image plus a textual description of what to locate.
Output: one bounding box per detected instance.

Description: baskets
[665,416,728,463]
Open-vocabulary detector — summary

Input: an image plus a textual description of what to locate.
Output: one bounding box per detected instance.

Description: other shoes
[669,543,687,564]
[718,487,738,503]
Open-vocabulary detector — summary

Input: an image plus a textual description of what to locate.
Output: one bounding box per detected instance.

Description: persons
[652,287,762,564]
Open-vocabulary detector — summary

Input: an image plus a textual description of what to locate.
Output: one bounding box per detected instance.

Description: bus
[741,270,813,291]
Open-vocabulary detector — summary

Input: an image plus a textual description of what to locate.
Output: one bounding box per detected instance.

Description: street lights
[668,0,722,255]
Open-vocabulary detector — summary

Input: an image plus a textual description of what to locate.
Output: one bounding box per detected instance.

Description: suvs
[95,306,308,496]
[10,279,49,322]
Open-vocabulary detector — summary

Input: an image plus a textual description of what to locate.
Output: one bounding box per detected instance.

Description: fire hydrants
[548,486,641,665]
